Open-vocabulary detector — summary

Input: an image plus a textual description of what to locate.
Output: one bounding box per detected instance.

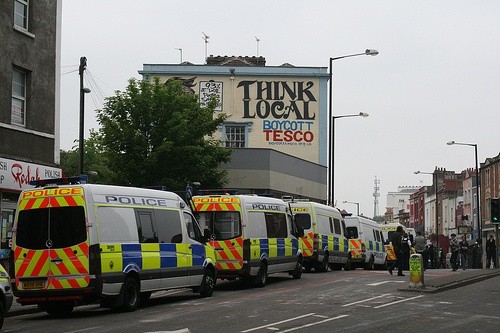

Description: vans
[342,216,388,270]
[277,198,350,274]
[380,221,416,268]
[6,174,214,321]
[185,192,305,289]
[0,264,15,331]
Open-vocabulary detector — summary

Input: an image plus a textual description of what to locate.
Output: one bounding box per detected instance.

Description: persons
[415,231,435,270]
[486,235,496,268]
[450,234,469,271]
[438,248,446,269]
[389,226,411,276]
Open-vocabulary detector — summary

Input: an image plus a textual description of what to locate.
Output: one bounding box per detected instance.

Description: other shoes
[397,272,405,276]
[388,267,393,275]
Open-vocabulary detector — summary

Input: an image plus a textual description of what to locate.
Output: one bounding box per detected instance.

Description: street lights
[414,170,439,251]
[446,140,481,240]
[343,200,360,216]
[328,48,379,207]
[331,111,370,209]
